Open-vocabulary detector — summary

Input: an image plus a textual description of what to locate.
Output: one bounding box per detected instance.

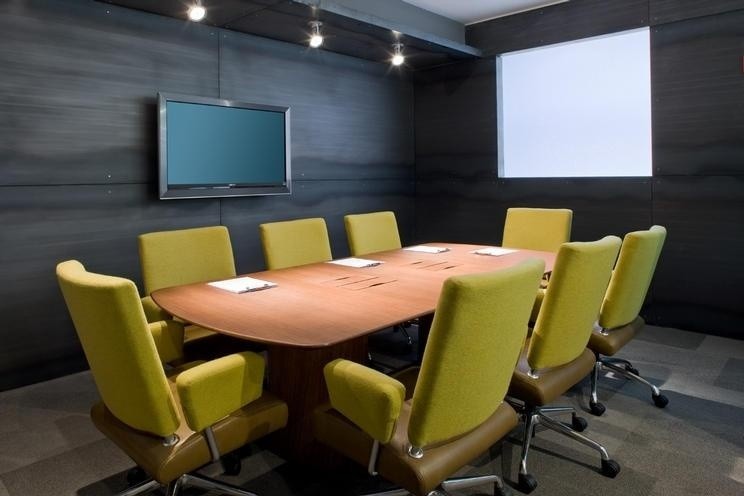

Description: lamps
[392,43,406,67]
[307,20,323,48]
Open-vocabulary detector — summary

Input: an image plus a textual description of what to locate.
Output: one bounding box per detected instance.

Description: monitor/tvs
[157,89,292,200]
